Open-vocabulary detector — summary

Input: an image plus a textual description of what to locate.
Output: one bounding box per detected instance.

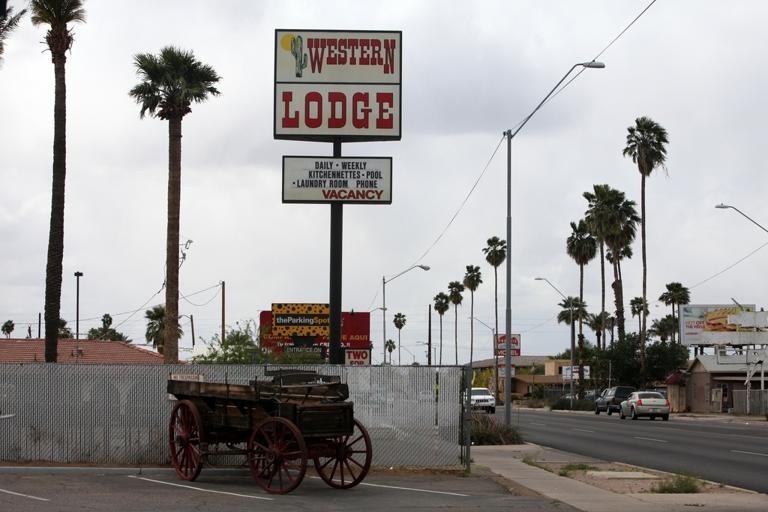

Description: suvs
[464,387,498,416]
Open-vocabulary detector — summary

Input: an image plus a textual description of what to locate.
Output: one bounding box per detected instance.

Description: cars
[559,384,674,422]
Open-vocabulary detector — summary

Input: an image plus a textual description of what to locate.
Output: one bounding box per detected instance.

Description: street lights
[504,61,607,429]
[713,202,768,234]
[398,333,439,365]
[368,306,388,315]
[381,262,433,366]
[532,276,576,411]
[464,314,497,360]
[74,271,84,339]
